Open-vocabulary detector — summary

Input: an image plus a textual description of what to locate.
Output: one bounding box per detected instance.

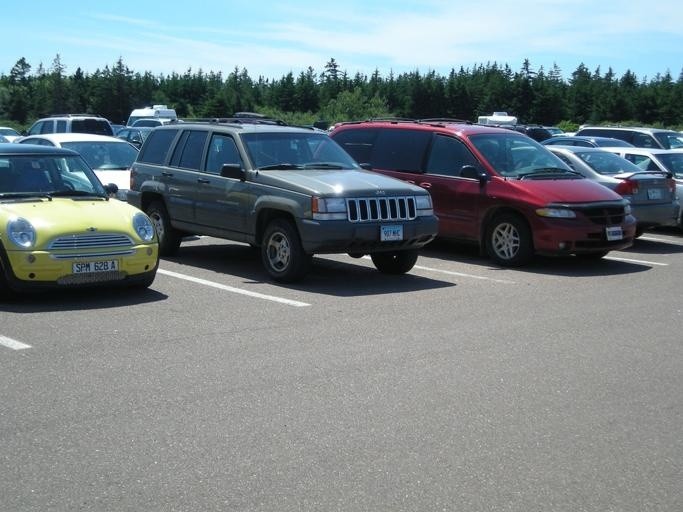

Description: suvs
[20,115,118,137]
[310,117,638,269]
[125,116,441,285]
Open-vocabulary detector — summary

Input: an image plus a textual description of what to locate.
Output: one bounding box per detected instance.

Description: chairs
[9,162,51,193]
[214,137,238,174]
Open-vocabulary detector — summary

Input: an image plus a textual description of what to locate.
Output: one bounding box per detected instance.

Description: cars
[0,99,683,231]
[12,132,140,205]
[0,141,160,299]
[508,144,682,242]
